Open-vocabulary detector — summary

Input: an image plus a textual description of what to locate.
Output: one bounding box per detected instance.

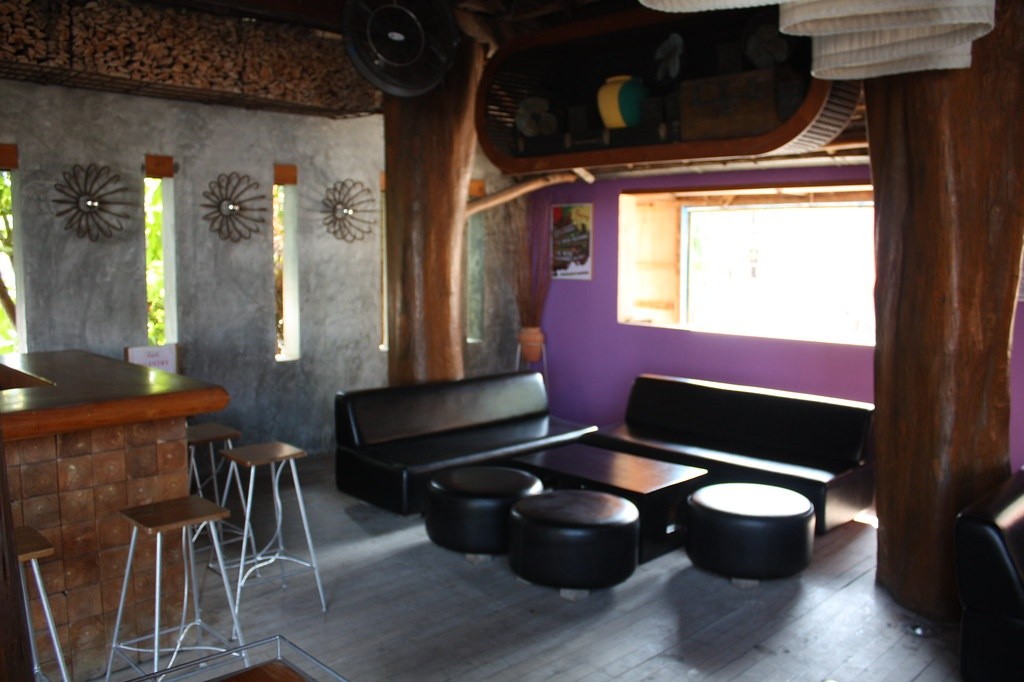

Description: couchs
[954,467,1024,682]
[335,370,598,517]
[580,374,875,537]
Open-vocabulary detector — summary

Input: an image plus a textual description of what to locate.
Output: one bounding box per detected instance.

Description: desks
[0,348,228,682]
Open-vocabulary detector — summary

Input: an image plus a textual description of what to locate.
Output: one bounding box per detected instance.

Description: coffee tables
[512,441,708,564]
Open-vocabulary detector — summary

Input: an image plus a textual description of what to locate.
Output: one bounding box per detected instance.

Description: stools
[12,423,327,681]
[426,466,544,561]
[507,490,639,600]
[686,482,816,588]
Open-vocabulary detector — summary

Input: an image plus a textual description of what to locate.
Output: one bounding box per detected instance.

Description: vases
[598,75,645,129]
[520,327,545,363]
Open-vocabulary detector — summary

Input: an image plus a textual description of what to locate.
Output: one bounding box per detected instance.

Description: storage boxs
[677,66,802,141]
